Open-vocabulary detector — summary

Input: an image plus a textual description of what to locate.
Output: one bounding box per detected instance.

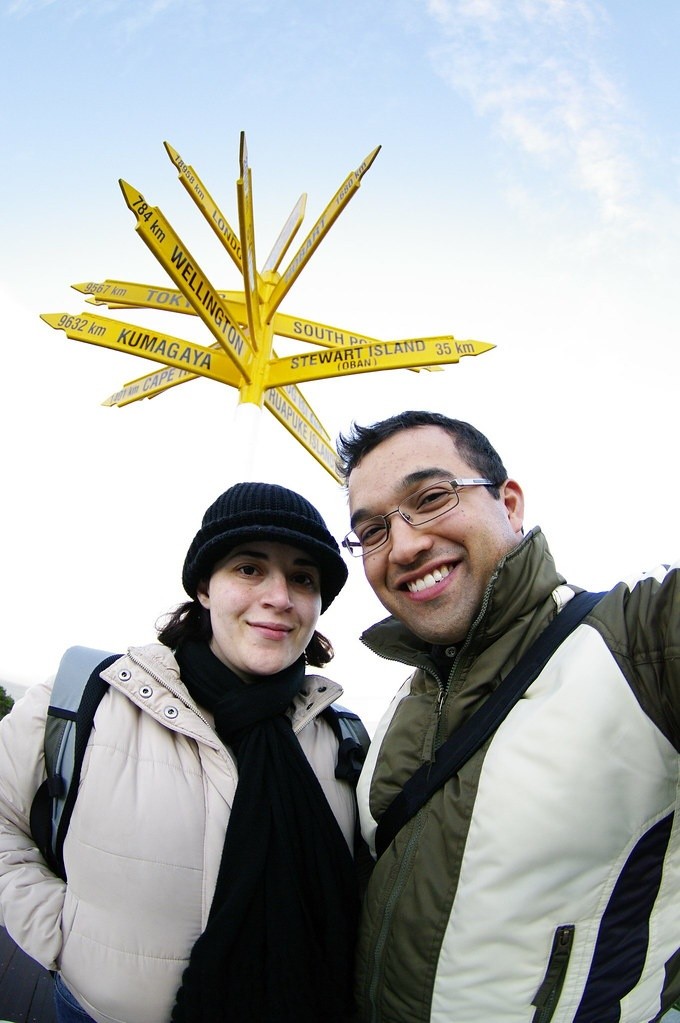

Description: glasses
[341,478,493,559]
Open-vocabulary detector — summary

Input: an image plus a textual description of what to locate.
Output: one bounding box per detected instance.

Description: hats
[180,483,349,615]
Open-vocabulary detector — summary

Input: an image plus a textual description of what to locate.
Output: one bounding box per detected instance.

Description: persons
[337,411,680,1023]
[0,483,376,1023]
